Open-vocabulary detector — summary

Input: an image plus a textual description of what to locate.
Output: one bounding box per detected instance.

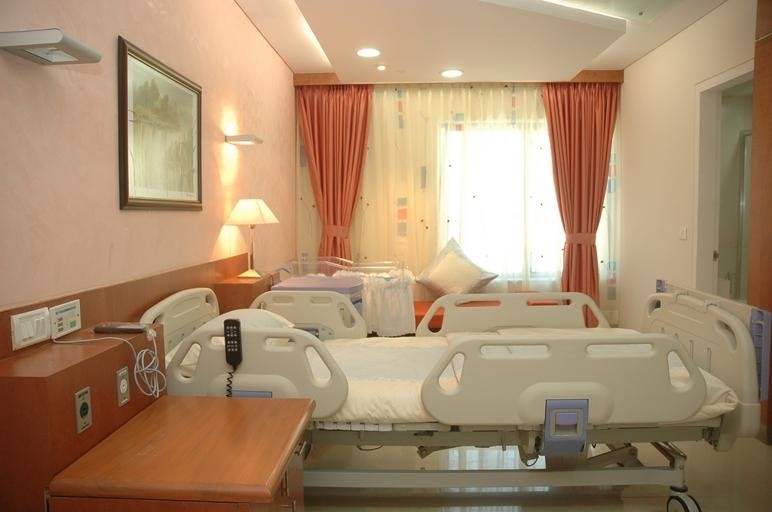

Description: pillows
[168,303,299,366]
[418,240,500,304]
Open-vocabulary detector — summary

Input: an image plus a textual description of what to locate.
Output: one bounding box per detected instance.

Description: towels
[362,272,416,338]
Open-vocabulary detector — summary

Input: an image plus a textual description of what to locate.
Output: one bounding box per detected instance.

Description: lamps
[1,27,102,70]
[227,198,278,280]
[226,133,265,148]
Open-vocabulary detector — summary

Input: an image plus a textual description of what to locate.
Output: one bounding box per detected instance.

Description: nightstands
[46,393,318,511]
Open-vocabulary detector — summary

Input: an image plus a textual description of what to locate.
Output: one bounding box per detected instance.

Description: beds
[133,284,755,512]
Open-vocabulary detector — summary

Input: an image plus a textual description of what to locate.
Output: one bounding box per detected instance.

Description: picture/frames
[116,31,204,212]
